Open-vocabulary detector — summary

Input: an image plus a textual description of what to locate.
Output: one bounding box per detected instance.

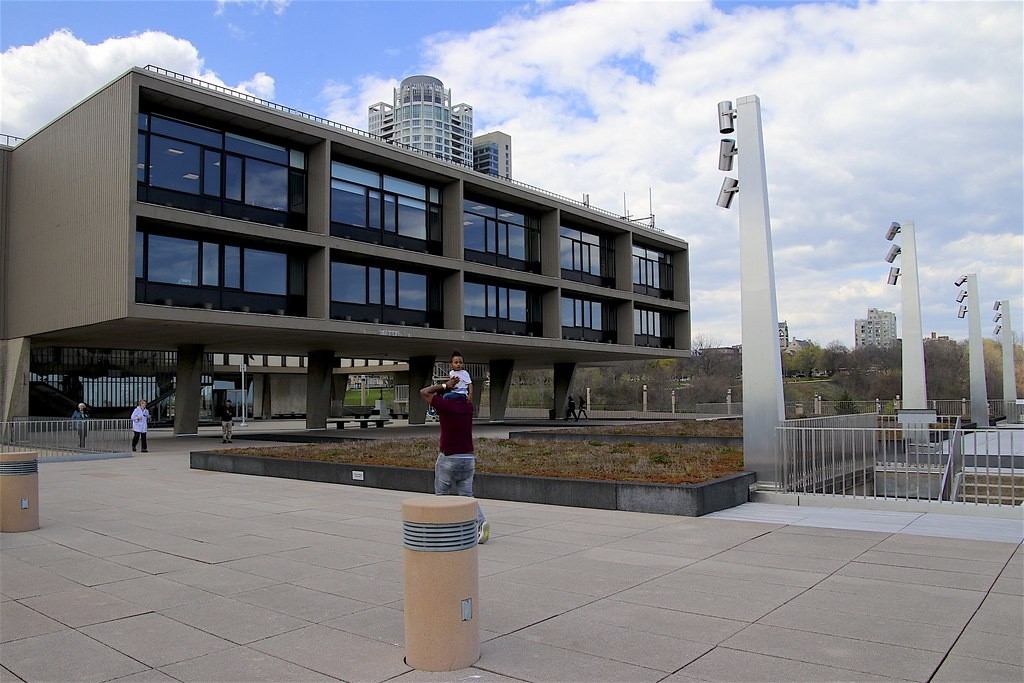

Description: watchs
[442,383,446,389]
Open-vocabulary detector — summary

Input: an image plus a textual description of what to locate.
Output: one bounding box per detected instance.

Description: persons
[564,396,578,422]
[427,410,433,415]
[221,400,233,443]
[146,402,168,421]
[71,403,90,448]
[420,376,490,544]
[577,396,588,422]
[442,352,473,403]
[131,399,149,453]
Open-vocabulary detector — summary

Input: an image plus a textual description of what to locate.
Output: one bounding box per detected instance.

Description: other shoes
[585,419,588,422]
[141,450,148,453]
[222,440,232,444]
[132,448,136,451]
[575,420,580,422]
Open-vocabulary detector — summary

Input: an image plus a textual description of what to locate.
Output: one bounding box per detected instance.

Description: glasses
[142,403,147,405]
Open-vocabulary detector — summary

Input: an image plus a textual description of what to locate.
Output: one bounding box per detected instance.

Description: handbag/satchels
[566,409,571,417]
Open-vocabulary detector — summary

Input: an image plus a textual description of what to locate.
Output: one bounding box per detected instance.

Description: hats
[227,400,232,403]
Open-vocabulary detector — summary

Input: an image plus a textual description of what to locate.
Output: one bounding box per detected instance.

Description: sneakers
[477,520,490,544]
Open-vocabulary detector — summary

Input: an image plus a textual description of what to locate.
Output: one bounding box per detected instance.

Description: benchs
[326,420,350,429]
[275,413,306,420]
[354,419,389,429]
